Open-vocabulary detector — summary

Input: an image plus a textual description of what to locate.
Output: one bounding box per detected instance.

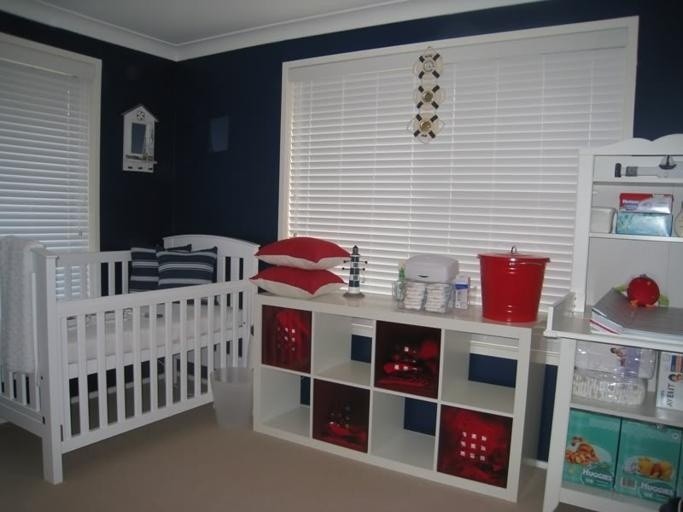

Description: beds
[1,232,260,486]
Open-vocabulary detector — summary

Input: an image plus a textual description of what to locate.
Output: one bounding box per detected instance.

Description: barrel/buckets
[476,243,550,325]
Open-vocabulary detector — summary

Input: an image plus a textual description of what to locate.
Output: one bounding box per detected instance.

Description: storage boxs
[613,417,680,506]
[561,404,624,495]
[671,460,680,505]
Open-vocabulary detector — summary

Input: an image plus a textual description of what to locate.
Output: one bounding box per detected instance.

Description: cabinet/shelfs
[541,134,682,512]
[251,289,546,504]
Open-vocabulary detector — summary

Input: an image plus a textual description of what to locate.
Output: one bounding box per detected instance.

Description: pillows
[253,237,353,270]
[155,245,216,302]
[245,265,347,299]
[129,243,191,295]
